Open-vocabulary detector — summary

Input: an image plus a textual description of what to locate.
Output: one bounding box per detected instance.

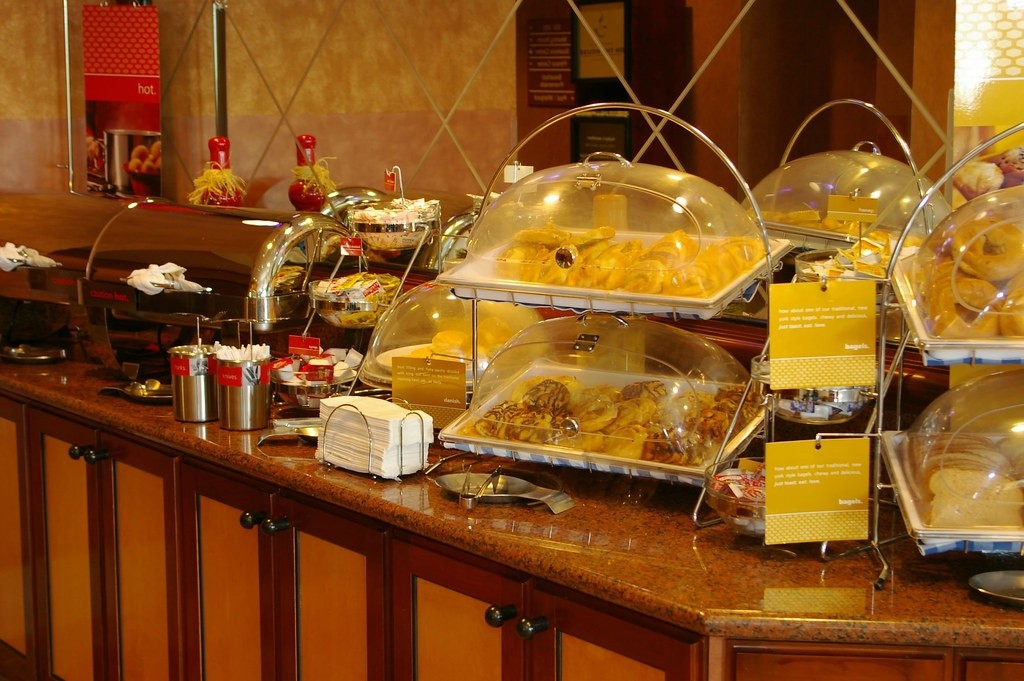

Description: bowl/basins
[122,163,161,196]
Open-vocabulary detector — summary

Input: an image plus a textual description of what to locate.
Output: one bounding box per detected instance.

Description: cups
[167,345,217,422]
[216,357,271,431]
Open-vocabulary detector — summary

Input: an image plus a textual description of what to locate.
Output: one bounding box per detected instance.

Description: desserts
[497,223,763,298]
[952,147,1024,201]
[458,372,758,465]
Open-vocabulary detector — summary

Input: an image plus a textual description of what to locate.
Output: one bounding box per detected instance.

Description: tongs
[458,464,502,509]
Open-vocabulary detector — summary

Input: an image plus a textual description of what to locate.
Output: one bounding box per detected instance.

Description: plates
[433,473,575,514]
[98,384,172,404]
[254,426,318,447]
[968,572,1024,610]
[0,344,65,363]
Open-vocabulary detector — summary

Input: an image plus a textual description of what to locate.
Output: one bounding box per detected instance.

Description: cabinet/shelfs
[0,298,1024,681]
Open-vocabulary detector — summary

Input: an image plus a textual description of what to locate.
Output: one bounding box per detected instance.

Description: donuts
[913,217,1024,340]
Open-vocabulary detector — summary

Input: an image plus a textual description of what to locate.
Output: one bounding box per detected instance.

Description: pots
[87,128,161,195]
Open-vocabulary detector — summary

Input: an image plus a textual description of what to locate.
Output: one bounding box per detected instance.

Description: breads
[405,329,490,367]
[902,428,1024,531]
